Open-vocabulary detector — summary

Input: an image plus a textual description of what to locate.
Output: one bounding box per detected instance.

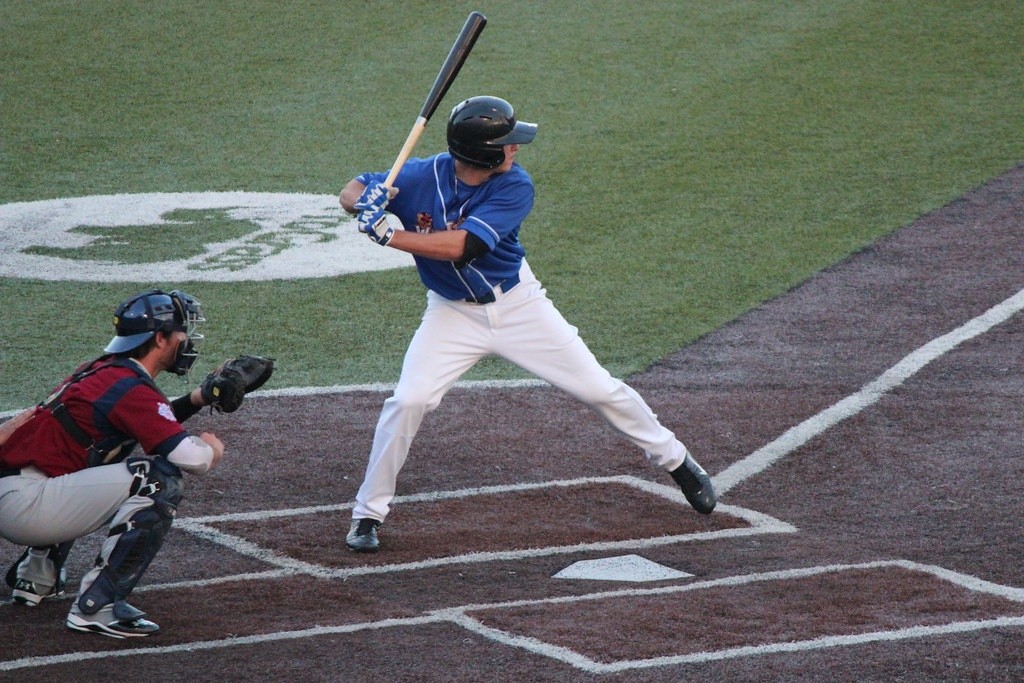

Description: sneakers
[346,518,380,553]
[13,578,65,607]
[667,451,716,515]
[66,600,161,640]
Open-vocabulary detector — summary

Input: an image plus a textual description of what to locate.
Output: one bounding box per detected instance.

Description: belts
[465,273,521,304]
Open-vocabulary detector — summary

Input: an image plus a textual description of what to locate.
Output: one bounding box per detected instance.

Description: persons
[341,95,715,556]
[0,292,274,640]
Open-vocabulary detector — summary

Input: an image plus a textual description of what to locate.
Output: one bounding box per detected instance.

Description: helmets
[104,288,207,375]
[445,97,538,171]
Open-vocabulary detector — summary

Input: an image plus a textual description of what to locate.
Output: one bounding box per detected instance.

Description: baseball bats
[379,12,491,188]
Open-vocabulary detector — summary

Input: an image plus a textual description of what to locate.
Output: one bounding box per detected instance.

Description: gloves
[353,205,394,248]
[358,180,398,208]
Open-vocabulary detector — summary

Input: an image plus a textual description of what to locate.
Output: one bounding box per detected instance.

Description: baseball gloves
[197,350,278,413]
